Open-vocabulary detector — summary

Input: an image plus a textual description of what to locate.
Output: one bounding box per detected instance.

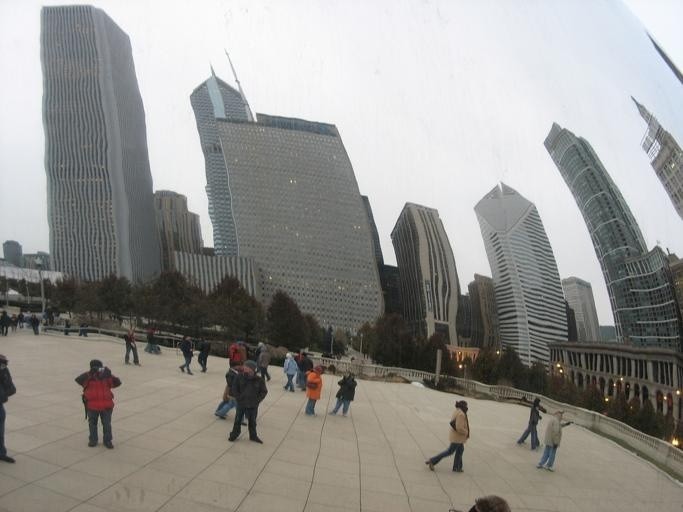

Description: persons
[535,410,573,472]
[227,361,267,444]
[213,339,312,426]
[0,354,16,463]
[73,360,121,448]
[304,367,323,415]
[447,496,509,512]
[0,311,88,338]
[329,374,356,416]
[423,400,469,473]
[515,397,544,450]
[123,329,140,366]
[144,326,160,355]
[197,335,208,372]
[178,335,193,375]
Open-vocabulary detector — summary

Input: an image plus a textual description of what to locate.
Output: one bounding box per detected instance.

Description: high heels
[425,459,435,471]
[453,467,464,472]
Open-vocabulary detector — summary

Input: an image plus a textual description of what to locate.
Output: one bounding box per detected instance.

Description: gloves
[90,366,112,379]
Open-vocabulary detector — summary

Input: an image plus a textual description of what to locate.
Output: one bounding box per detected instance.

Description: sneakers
[215,412,263,444]
[0,455,15,463]
[536,464,554,472]
[88,439,113,449]
[263,377,306,392]
[179,365,207,375]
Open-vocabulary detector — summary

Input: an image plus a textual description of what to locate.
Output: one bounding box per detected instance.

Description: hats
[243,360,257,371]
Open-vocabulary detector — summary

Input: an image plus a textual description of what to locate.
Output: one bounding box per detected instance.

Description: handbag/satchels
[306,382,318,389]
[450,418,456,431]
